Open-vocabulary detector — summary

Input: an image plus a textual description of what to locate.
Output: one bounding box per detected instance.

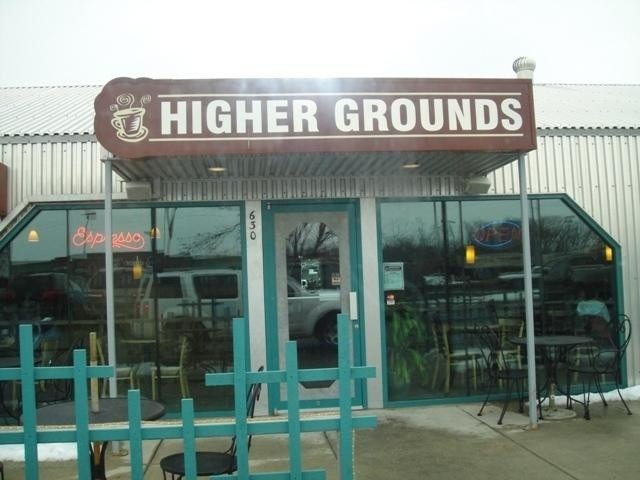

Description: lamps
[464,173,492,197]
[124,179,156,199]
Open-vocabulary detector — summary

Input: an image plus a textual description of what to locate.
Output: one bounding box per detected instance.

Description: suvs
[135,264,346,363]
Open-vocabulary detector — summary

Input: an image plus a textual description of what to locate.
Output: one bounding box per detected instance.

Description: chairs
[84,326,267,479]
[431,311,637,428]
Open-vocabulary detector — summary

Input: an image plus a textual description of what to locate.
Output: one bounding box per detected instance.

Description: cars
[80,267,147,320]
[20,271,84,320]
[498,265,553,288]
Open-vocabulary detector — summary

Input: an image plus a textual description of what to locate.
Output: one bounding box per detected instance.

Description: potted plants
[382,307,427,398]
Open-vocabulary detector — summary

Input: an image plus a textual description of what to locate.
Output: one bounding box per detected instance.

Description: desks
[19,400,166,480]
[0,353,42,424]
